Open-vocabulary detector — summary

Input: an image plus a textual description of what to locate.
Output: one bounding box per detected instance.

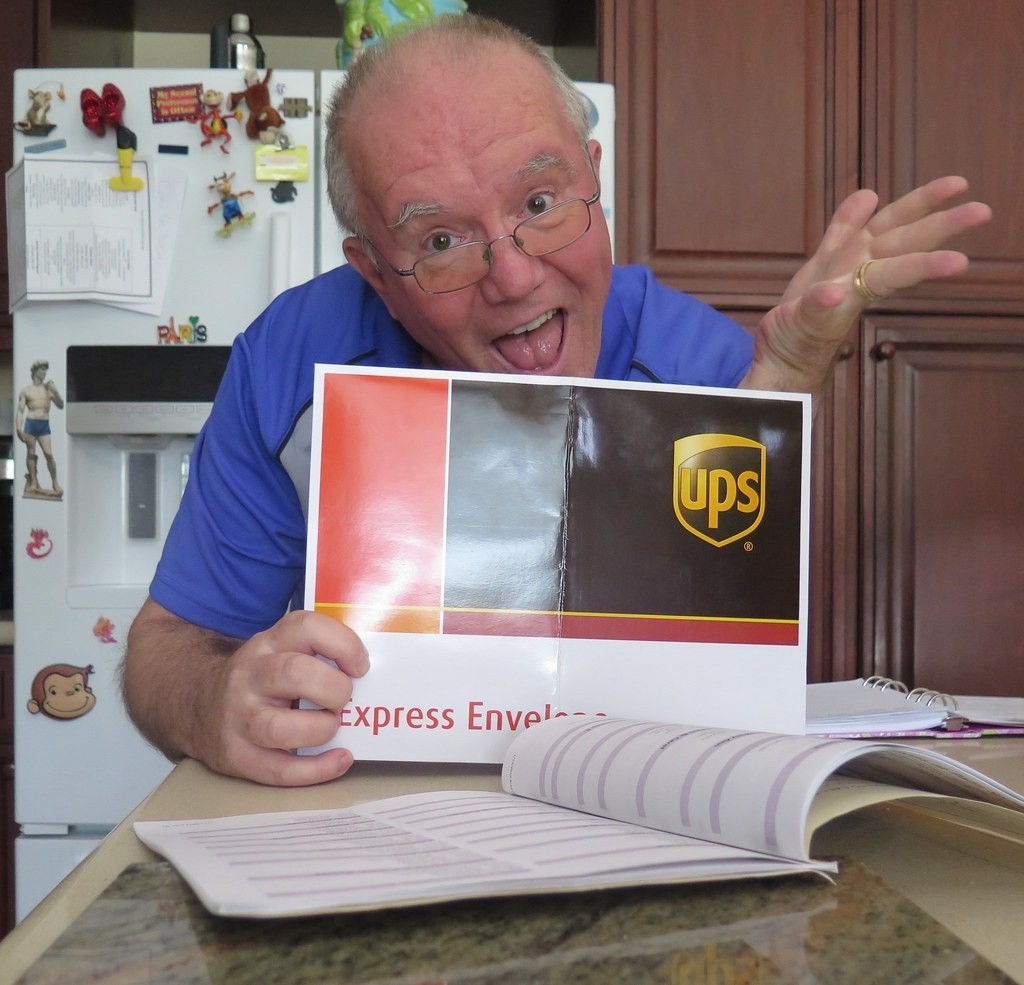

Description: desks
[1,739,1024,985]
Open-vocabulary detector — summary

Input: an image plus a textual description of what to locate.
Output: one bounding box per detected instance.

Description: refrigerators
[13,69,615,927]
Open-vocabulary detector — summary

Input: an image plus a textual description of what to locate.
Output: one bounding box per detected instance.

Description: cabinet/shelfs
[598,0,1024,698]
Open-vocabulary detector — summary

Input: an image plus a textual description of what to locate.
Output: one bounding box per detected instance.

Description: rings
[853,259,885,302]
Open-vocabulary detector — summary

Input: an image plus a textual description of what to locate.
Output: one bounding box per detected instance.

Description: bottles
[230,14,263,68]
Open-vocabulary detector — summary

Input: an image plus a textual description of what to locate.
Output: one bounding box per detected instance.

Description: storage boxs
[114,30,211,69]
[256,36,340,73]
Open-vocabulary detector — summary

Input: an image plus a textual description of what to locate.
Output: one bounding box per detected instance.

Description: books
[130,716,1024,919]
[806,675,1024,742]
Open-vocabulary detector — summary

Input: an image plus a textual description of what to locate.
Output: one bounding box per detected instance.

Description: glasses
[359,144,600,294]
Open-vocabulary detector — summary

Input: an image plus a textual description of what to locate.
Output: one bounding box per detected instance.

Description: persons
[16,360,64,495]
[122,18,993,786]
